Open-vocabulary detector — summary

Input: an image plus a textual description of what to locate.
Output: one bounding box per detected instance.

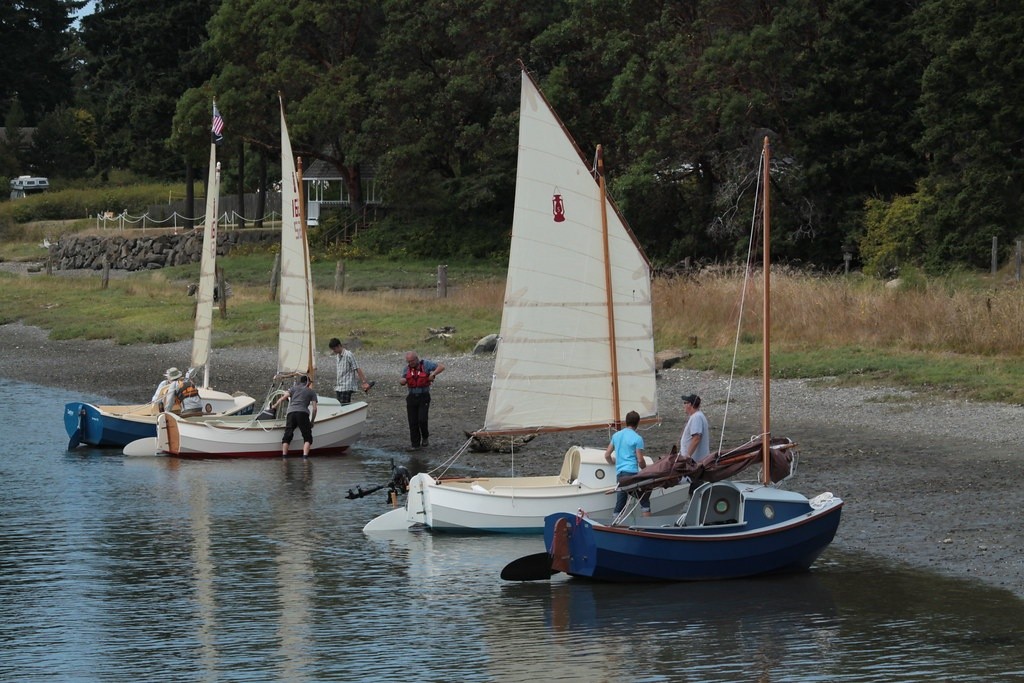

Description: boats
[10,175,50,191]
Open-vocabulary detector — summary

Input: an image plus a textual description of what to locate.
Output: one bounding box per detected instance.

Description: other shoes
[409,446,420,452]
[422,438,428,447]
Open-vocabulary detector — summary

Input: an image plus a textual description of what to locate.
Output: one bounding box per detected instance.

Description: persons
[604,410,651,524]
[150,367,204,420]
[680,394,710,467]
[399,350,444,451]
[280,458,313,501]
[328,338,370,406]
[271,376,318,461]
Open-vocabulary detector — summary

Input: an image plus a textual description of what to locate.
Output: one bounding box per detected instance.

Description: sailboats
[541,134,844,581]
[402,56,695,536]
[62,140,257,450]
[157,87,370,458]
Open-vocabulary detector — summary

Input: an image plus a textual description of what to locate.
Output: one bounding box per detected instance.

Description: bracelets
[430,372,435,379]
[361,380,366,385]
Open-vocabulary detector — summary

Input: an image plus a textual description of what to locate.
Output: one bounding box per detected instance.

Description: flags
[210,99,225,144]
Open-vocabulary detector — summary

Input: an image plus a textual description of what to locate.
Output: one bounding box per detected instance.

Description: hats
[164,366,183,380]
[682,394,701,408]
[301,375,314,383]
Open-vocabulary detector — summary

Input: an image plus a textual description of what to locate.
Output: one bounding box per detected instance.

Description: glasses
[408,356,417,363]
[683,401,690,404]
